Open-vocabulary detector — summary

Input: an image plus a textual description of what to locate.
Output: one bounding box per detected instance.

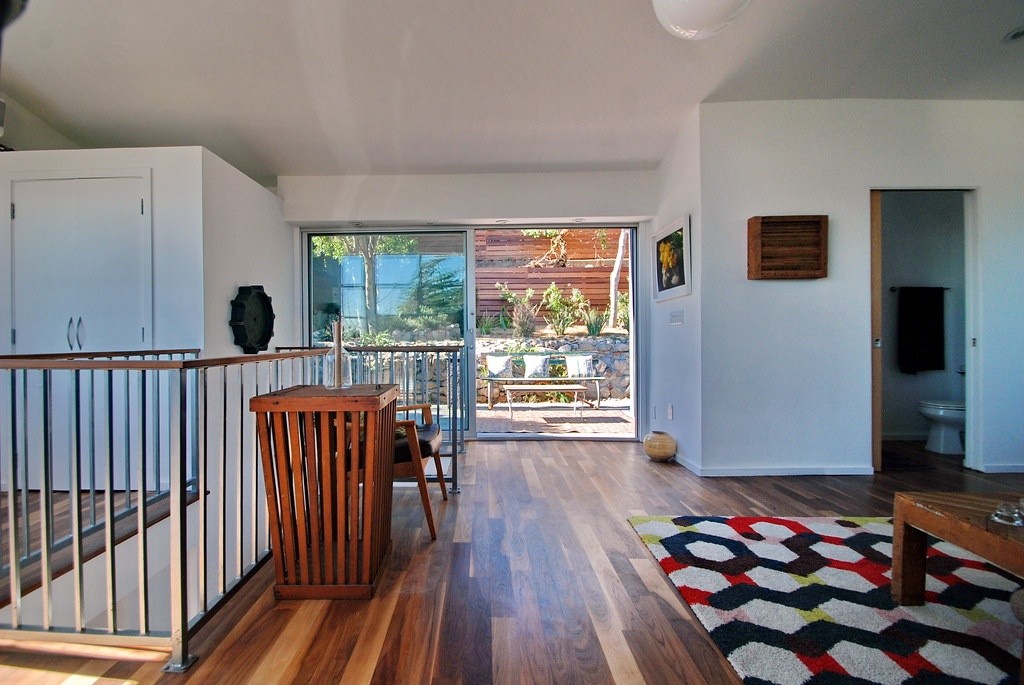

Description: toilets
[915,366,966,455]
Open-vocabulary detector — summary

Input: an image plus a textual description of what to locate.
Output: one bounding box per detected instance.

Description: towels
[892,285,945,375]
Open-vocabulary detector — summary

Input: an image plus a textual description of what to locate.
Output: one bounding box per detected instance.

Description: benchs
[481,352,606,410]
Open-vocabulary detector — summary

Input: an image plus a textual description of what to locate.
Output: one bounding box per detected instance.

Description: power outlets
[667,404,673,419]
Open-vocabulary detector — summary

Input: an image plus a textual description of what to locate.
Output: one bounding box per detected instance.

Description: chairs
[333,403,449,539]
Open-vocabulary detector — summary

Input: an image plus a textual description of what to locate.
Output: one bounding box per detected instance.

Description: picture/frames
[650,213,692,301]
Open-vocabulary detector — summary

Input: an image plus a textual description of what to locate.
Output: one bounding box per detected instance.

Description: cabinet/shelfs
[748,215,828,279]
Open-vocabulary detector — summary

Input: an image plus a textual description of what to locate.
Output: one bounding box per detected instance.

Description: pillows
[523,355,550,378]
[565,355,594,378]
[486,355,514,378]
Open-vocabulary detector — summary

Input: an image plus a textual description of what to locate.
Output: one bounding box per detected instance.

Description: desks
[503,384,588,422]
[890,492,1024,607]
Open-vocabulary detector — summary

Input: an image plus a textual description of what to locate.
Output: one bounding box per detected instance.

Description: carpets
[627,515,1024,685]
